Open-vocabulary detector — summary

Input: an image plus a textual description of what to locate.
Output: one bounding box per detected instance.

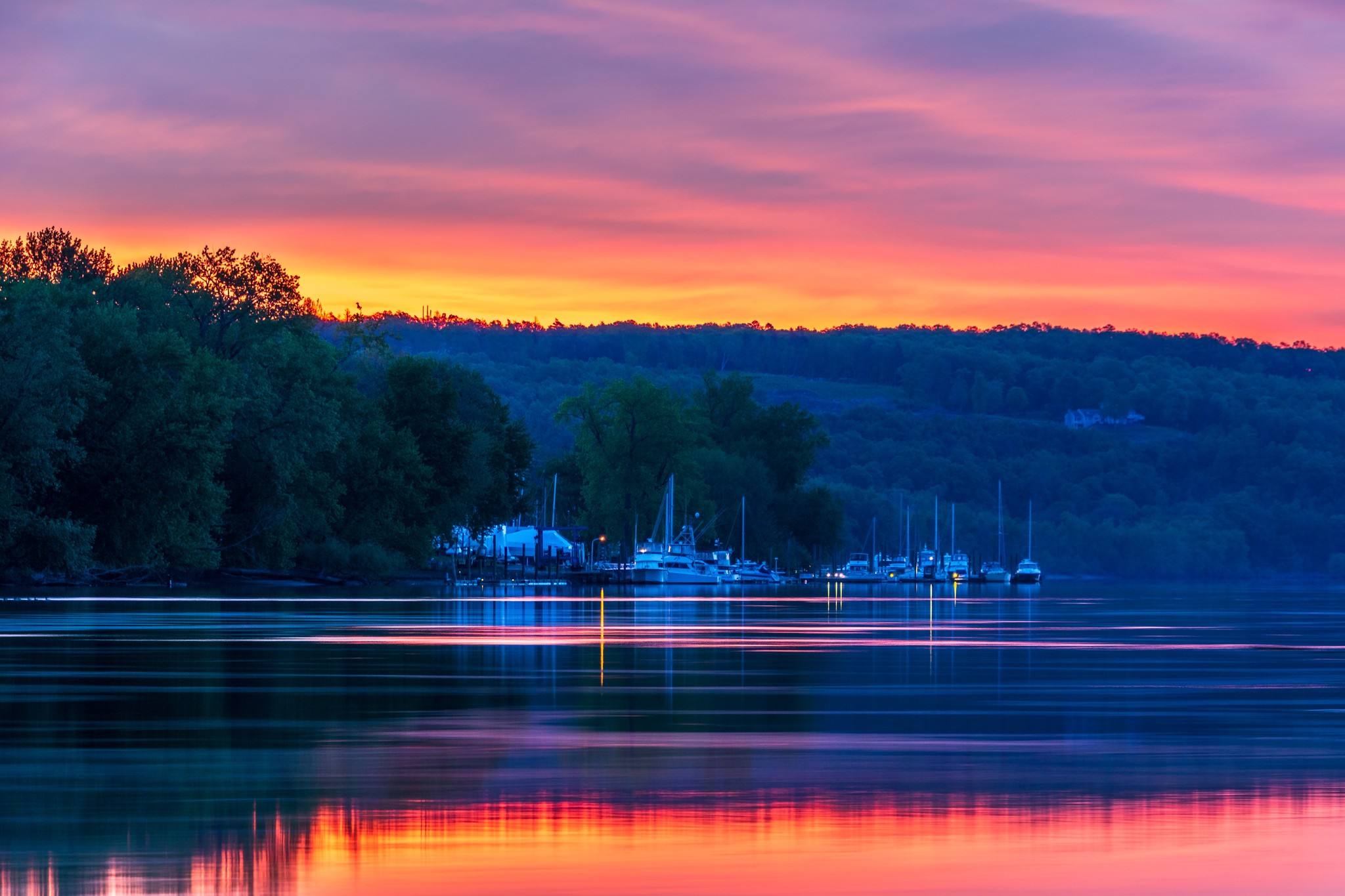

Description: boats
[430,468,1044,588]
